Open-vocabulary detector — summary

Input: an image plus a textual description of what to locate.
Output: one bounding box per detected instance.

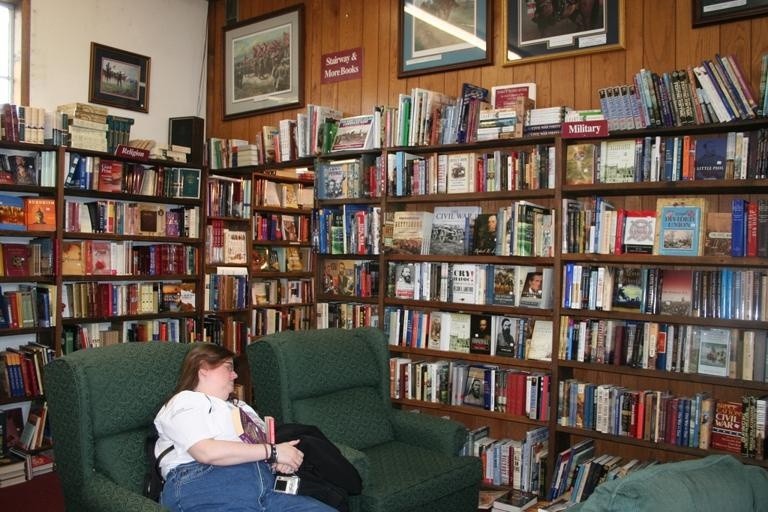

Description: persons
[465,379,484,406]
[154,344,340,511]
[472,319,489,349]
[338,262,353,298]
[497,319,514,354]
[394,268,414,299]
[524,273,542,301]
[481,215,497,252]
[34,211,45,226]
[324,180,340,196]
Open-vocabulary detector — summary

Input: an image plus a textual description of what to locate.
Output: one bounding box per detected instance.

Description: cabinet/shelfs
[319,118,768,512]
[0,144,202,463]
[204,157,317,353]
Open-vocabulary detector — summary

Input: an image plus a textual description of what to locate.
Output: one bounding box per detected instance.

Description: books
[318,153,385,200]
[66,197,200,240]
[0,192,57,233]
[253,213,314,240]
[264,416,275,444]
[206,269,250,312]
[389,146,558,194]
[65,321,192,352]
[558,376,766,462]
[254,246,314,273]
[386,356,549,422]
[206,174,249,217]
[558,316,766,381]
[318,259,380,298]
[560,196,767,256]
[233,407,265,442]
[523,53,767,132]
[463,426,551,512]
[561,263,766,319]
[384,306,552,362]
[253,308,310,336]
[1,101,190,163]
[1,237,54,275]
[0,455,54,487]
[386,260,553,309]
[2,402,49,453]
[210,103,342,168]
[264,166,316,178]
[256,178,314,211]
[564,132,767,183]
[331,82,536,151]
[315,204,382,255]
[65,152,202,199]
[203,313,250,354]
[64,282,195,319]
[64,238,198,277]
[2,341,55,398]
[383,199,555,256]
[0,284,57,329]
[1,152,56,185]
[317,301,378,329]
[254,278,313,306]
[553,439,663,507]
[204,220,248,266]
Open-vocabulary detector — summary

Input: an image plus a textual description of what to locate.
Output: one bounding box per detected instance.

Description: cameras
[273,476,299,495]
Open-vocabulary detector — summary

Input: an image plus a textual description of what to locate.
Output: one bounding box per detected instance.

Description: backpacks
[143,431,178,501]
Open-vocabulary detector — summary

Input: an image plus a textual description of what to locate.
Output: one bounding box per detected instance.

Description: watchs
[267,442,276,466]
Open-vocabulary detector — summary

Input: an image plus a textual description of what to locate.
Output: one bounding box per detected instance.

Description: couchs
[246,328,483,511]
[554,453,764,510]
[41,342,222,512]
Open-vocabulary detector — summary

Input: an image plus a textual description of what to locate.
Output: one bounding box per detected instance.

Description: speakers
[167,116,203,173]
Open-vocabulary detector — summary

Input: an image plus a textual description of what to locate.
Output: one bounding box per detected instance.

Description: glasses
[207,358,234,373]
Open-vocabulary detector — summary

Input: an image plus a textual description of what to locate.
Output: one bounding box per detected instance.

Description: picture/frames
[88,42,151,113]
[501,0,626,68]
[397,1,494,79]
[220,4,304,122]
[691,1,767,29]
